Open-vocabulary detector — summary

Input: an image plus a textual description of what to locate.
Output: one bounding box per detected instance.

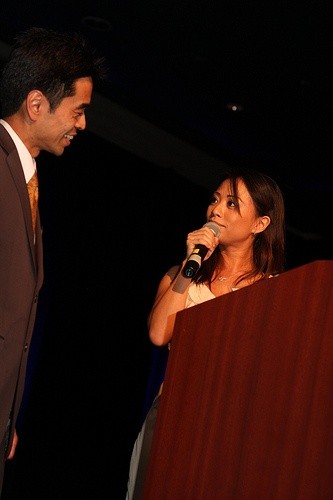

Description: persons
[125,165,285,500]
[0,28,104,484]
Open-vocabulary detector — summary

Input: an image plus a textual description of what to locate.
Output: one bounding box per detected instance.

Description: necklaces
[214,260,255,281]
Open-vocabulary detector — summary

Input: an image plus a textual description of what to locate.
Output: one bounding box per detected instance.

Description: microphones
[182,222,220,278]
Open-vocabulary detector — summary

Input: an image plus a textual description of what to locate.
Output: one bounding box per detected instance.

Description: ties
[25,171,38,237]
